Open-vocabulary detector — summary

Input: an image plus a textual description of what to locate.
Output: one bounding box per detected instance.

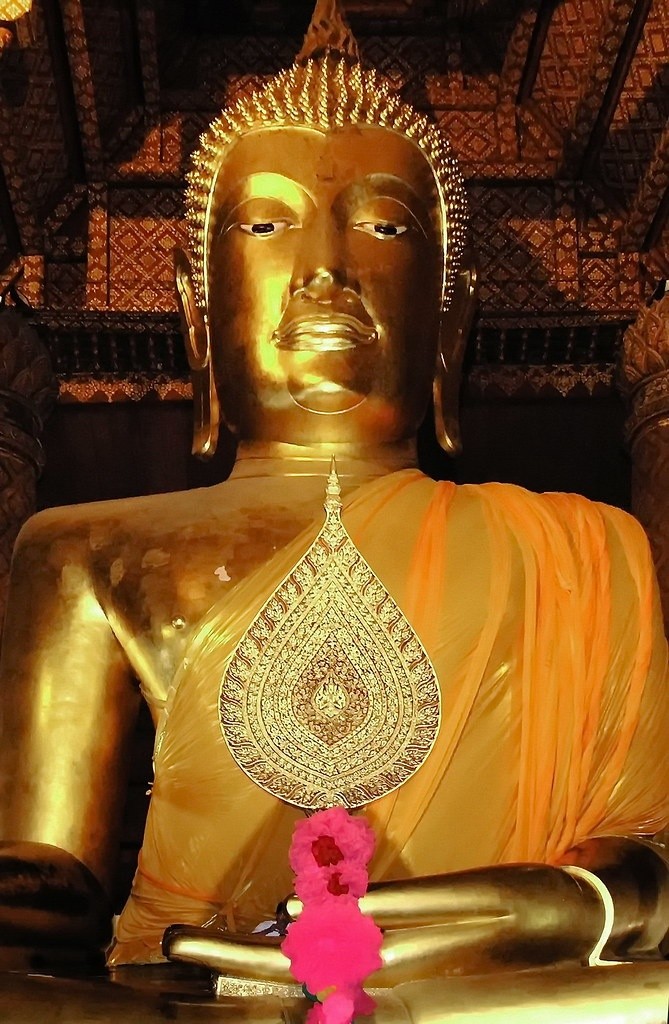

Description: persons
[1,0,668,1023]
